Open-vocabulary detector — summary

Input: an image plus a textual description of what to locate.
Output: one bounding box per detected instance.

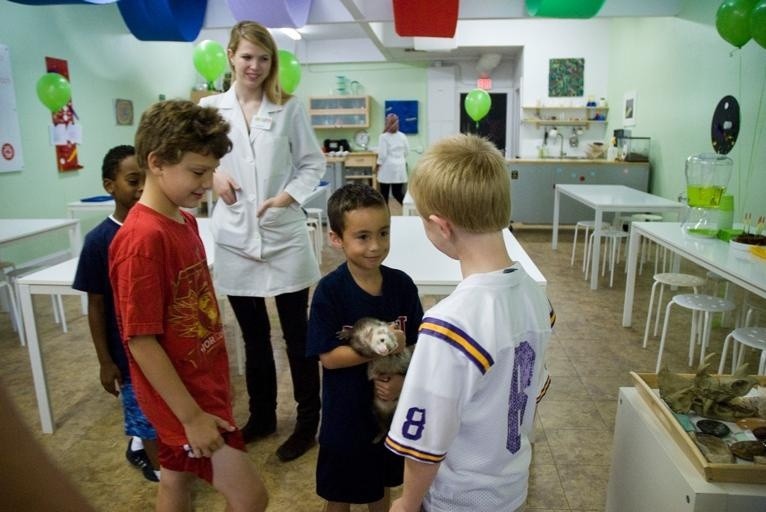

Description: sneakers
[126,438,161,482]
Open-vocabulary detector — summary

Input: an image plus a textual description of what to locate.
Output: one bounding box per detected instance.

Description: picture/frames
[621,89,639,129]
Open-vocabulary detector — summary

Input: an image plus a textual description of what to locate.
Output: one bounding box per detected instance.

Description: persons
[301,181,425,511]
[381,132,561,511]
[196,20,328,463]
[105,98,272,512]
[69,146,159,480]
[376,111,410,204]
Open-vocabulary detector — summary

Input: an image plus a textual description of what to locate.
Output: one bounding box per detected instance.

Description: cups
[718,195,735,232]
[350,81,359,95]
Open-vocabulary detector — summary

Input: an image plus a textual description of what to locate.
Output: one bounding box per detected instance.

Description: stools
[655,293,738,376]
[734,287,765,371]
[584,229,629,288]
[717,327,765,376]
[624,214,663,263]
[642,273,707,349]
[571,221,613,271]
[49,294,69,335]
[705,270,741,330]
[1,261,21,290]
[301,207,324,266]
[0,279,27,347]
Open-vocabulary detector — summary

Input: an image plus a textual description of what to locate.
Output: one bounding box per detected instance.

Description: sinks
[540,155,584,160]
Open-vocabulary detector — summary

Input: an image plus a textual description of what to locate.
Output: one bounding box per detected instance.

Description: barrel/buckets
[680,154,733,236]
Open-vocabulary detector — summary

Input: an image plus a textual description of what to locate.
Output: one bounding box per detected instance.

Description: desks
[551,182,687,292]
[380,215,548,301]
[17,217,245,437]
[0,217,89,317]
[300,182,331,240]
[66,196,126,258]
[322,149,380,192]
[622,221,764,330]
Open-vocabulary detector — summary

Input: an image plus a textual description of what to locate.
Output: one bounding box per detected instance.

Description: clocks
[354,130,370,147]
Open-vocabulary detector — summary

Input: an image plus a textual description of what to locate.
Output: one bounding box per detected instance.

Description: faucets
[557,133,567,156]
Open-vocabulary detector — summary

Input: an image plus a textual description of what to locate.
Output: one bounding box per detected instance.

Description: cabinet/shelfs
[602,385,765,512]
[521,102,611,130]
[508,158,655,232]
[306,96,370,130]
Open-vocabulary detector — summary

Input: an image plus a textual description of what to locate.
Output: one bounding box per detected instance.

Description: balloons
[192,39,228,90]
[715,1,753,50]
[276,49,303,95]
[744,3,766,54]
[34,71,75,116]
[464,89,493,129]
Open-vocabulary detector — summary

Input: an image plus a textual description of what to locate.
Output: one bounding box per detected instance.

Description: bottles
[223,72,231,92]
[336,75,345,95]
[587,102,597,120]
[608,139,618,161]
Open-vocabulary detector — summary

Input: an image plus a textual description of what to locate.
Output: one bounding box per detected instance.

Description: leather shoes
[239,410,277,443]
[275,433,316,461]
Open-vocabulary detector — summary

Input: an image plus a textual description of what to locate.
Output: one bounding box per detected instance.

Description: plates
[697,420,730,437]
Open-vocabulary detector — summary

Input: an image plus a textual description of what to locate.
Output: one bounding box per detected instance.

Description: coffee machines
[613,129,631,159]
